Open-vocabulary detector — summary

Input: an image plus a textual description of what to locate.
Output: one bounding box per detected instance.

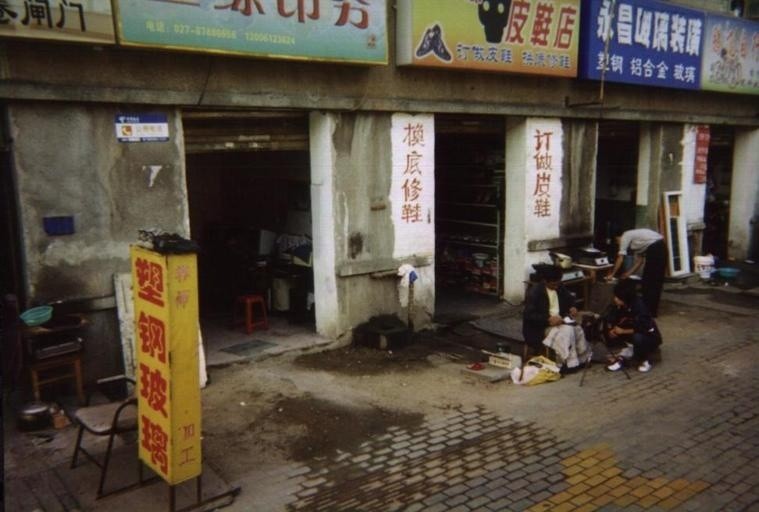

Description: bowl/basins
[19,305,53,327]
[718,267,740,278]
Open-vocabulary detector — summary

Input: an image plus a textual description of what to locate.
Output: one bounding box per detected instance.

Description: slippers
[467,363,484,369]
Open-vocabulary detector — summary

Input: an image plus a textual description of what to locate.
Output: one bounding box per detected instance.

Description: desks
[523,276,591,311]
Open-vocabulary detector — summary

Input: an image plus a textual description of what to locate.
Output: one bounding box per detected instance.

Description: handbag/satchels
[640,316,662,351]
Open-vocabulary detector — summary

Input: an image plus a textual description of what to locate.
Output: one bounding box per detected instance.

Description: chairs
[70,374,163,500]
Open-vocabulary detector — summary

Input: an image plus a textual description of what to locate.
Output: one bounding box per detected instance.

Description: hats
[615,278,635,304]
[543,266,562,281]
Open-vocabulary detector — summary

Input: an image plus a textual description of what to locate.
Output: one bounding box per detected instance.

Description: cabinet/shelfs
[435,180,505,303]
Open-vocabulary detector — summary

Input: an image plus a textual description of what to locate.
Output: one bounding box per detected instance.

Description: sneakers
[637,361,652,371]
[561,364,576,373]
[138,227,198,251]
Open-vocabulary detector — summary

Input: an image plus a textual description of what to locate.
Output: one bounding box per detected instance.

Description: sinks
[370,321,405,351]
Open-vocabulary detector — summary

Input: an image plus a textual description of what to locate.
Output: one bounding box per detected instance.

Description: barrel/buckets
[693,255,715,280]
[472,252,489,269]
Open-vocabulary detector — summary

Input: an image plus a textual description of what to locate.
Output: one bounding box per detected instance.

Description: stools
[522,342,552,370]
[29,351,88,406]
[232,295,268,335]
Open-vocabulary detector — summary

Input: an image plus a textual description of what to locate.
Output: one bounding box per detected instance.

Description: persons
[520,264,591,375]
[590,279,664,374]
[604,226,668,320]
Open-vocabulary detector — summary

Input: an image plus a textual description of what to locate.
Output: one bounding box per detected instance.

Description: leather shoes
[433,36,451,60]
[416,25,441,55]
